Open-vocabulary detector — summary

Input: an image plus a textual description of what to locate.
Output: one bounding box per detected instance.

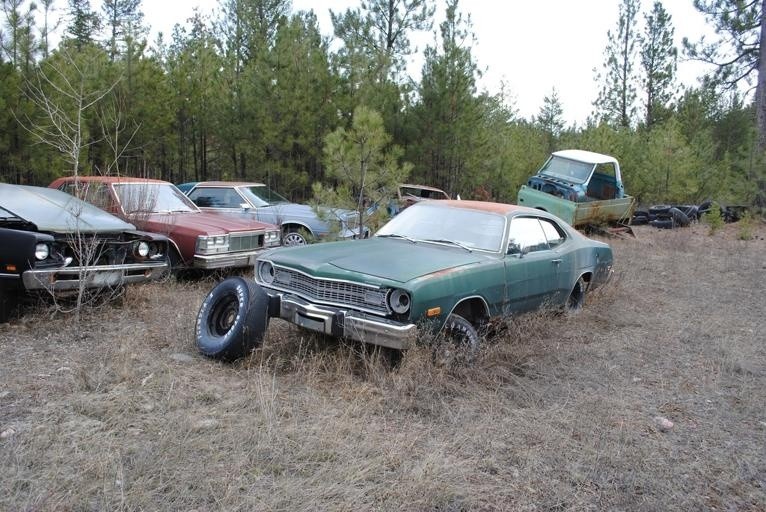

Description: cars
[176,181,370,248]
[46,175,282,273]
[254,198,613,367]
[0,172,170,324]
